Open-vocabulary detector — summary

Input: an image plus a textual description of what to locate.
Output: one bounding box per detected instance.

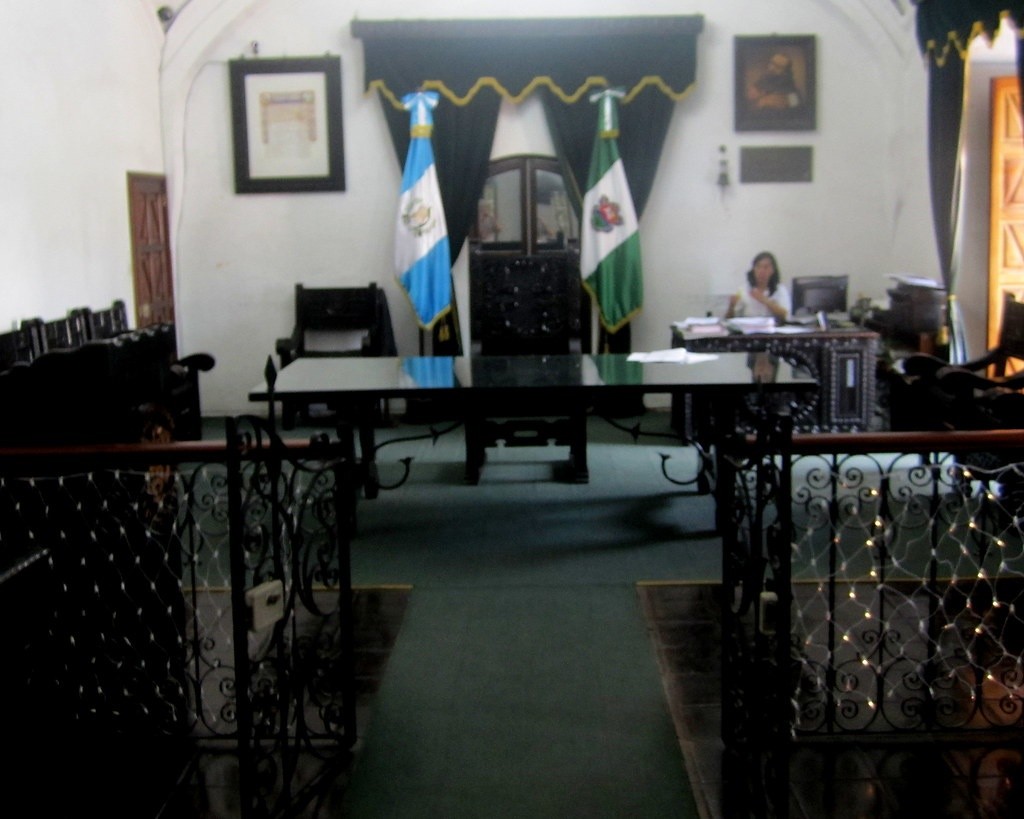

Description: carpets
[635,579,1024,819]
[178,584,411,819]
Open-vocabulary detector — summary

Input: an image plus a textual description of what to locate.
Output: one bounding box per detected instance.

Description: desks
[248,353,821,544]
[668,318,882,449]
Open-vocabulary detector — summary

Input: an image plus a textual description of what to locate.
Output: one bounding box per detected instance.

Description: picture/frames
[732,32,818,133]
[227,55,346,196]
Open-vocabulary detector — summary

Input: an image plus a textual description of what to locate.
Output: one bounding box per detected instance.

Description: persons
[747,51,802,109]
[726,251,794,321]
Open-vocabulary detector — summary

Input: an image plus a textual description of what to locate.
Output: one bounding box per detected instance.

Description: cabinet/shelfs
[464,245,590,485]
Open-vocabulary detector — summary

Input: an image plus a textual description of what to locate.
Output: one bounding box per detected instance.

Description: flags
[578,86,645,336]
[388,92,455,332]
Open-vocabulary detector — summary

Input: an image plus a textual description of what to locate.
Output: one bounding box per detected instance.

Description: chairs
[889,292,1024,483]
[1,300,216,472]
[276,282,400,432]
[790,273,850,322]
